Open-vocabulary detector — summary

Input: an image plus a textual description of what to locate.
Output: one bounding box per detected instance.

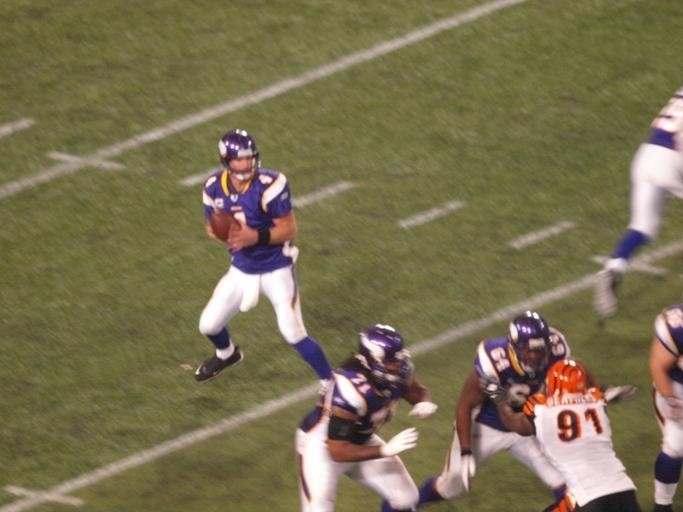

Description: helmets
[360,324,414,391]
[218,130,258,180]
[508,311,586,394]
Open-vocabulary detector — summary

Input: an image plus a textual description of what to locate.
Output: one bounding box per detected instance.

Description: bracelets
[255,227,271,247]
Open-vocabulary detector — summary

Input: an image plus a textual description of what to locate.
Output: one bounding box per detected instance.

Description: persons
[294,324,438,511]
[478,358,642,512]
[192,131,333,389]
[382,308,568,511]
[589,85,683,317]
[647,306,683,512]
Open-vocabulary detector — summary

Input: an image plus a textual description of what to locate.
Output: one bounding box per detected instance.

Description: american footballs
[210,212,242,240]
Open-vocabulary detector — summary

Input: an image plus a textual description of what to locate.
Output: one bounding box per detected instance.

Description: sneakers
[194,346,244,383]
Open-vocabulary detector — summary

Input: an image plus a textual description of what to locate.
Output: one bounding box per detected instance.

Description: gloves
[380,427,419,455]
[480,375,505,401]
[408,401,438,418]
[462,453,476,491]
